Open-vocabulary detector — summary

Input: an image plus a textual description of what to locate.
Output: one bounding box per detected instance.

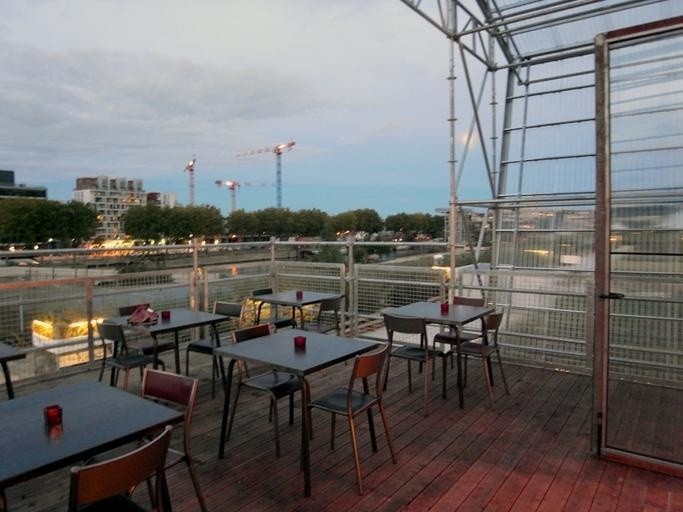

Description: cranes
[214,179,274,213]
[233,139,294,209]
[182,158,197,206]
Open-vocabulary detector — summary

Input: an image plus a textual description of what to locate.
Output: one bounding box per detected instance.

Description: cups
[162,310,171,319]
[44,404,63,426]
[296,290,303,300]
[295,336,306,350]
[441,303,449,315]
[45,423,63,442]
